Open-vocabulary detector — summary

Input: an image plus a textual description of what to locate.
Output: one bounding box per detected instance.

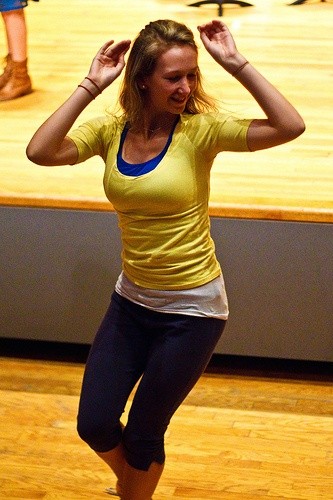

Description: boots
[0,54,31,101]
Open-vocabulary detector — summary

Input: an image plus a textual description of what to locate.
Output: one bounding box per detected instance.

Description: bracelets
[77,76,101,100]
[230,60,248,78]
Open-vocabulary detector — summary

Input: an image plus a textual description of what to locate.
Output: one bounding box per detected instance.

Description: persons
[0,0,36,103]
[23,22,306,499]
